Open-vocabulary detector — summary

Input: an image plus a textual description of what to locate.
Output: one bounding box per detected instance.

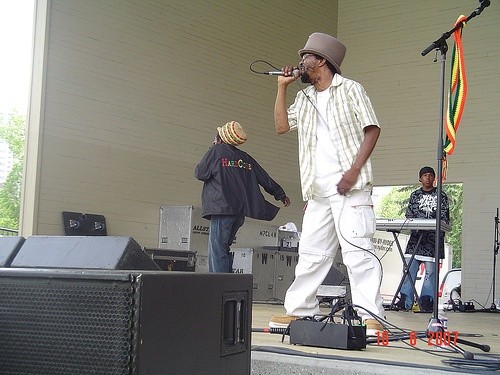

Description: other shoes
[362,319,384,337]
[397,293,407,309]
[269,314,300,328]
[419,295,433,312]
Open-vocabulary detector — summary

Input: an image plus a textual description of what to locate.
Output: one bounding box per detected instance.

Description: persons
[268,32,385,338]
[194,121,291,272]
[395,166,450,311]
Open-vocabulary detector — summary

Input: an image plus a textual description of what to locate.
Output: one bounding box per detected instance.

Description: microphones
[264,70,300,76]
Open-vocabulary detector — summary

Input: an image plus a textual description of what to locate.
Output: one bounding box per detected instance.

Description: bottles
[436,308,448,333]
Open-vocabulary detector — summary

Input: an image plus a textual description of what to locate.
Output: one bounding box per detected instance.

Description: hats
[419,166,435,178]
[217,120,248,147]
[298,32,347,75]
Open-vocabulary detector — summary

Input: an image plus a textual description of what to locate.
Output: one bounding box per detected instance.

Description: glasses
[302,54,315,61]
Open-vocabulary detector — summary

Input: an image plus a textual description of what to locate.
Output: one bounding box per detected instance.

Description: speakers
[0,234,253,375]
[321,266,345,286]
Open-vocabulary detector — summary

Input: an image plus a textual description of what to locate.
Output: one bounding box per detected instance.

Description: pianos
[375,218,452,314]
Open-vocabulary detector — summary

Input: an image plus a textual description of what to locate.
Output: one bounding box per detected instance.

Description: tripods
[366,0,491,359]
[464,208,500,314]
